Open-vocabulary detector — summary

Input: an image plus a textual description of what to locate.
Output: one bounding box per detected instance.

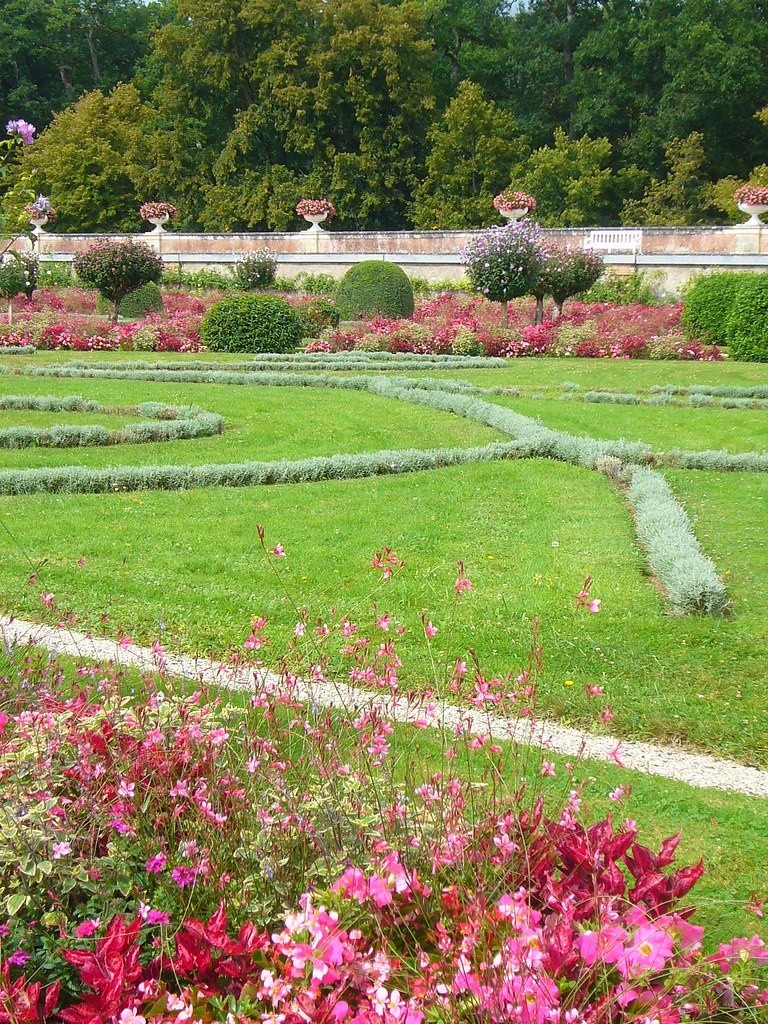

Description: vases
[499,207,528,228]
[303,212,328,232]
[148,211,169,232]
[29,215,49,232]
[737,202,768,225]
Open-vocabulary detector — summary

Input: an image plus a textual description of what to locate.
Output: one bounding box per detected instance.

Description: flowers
[732,184,768,206]
[139,201,179,220]
[20,205,58,223]
[492,190,537,215]
[296,198,335,224]
